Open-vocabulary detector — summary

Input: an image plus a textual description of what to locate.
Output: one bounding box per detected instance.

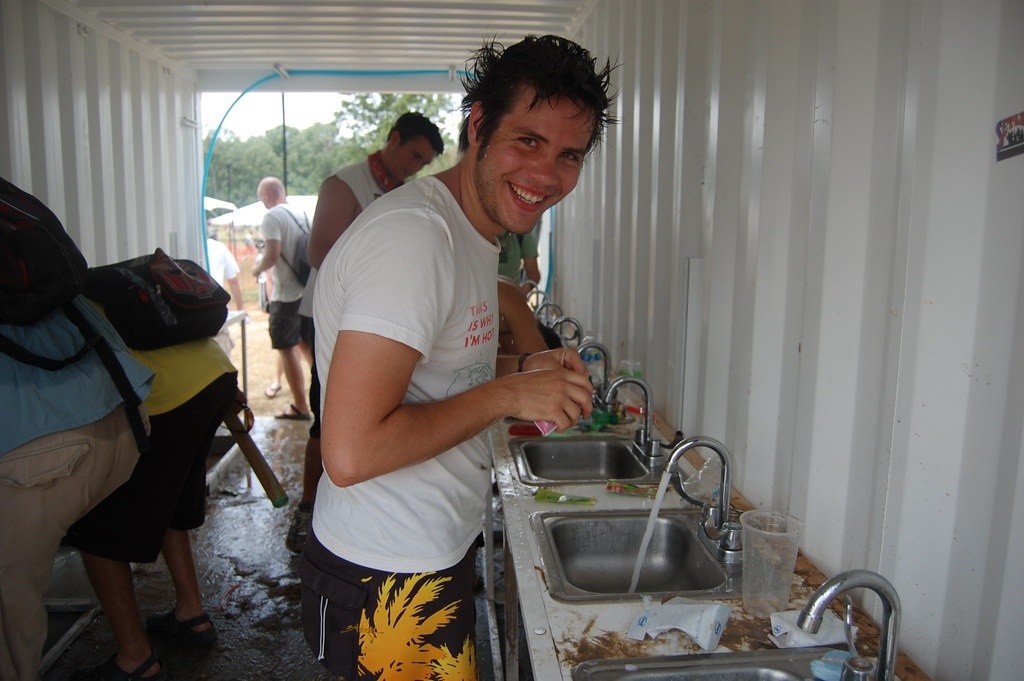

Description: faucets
[796,568,902,681]
[526,290,550,303]
[664,434,731,531]
[605,374,656,442]
[551,316,583,345]
[519,280,538,292]
[577,342,612,383]
[535,303,564,319]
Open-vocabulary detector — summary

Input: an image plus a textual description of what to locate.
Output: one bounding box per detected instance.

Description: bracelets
[518,352,531,372]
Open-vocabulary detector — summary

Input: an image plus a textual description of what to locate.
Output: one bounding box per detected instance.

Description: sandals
[146,606,215,645]
[81,649,161,681]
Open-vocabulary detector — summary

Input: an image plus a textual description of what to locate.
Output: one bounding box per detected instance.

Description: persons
[498,226,546,356]
[204,211,242,312]
[301,33,624,680]
[255,178,311,419]
[296,113,444,523]
[0,300,156,681]
[244,230,254,253]
[62,338,238,681]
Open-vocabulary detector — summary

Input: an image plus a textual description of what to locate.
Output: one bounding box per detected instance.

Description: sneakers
[287,508,312,552]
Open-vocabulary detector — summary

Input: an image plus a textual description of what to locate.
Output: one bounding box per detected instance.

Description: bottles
[579,329,603,386]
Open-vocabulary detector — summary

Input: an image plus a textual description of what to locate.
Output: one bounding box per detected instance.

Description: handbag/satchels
[0,176,87,326]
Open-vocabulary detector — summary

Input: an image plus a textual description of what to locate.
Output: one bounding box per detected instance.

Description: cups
[618,358,644,407]
[739,508,806,619]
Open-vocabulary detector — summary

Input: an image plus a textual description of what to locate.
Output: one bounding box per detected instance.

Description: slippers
[265,383,281,399]
[275,403,311,420]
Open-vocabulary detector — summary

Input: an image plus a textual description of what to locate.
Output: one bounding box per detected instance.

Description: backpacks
[84,246,229,350]
[278,206,315,288]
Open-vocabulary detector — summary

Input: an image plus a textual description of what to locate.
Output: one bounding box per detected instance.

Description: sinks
[591,665,801,681]
[549,516,729,593]
[520,440,652,480]
